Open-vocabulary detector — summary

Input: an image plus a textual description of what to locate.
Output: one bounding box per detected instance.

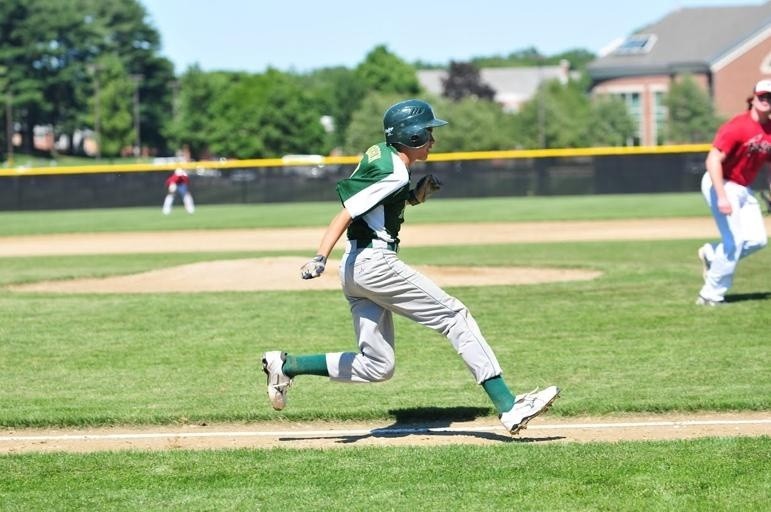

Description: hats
[754,80,771,96]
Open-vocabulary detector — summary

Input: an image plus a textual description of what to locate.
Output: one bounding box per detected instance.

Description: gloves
[407,175,444,207]
[299,255,328,280]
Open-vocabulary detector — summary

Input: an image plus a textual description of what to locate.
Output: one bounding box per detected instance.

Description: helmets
[383,100,448,149]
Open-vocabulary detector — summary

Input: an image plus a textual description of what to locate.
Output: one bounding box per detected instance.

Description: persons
[261,100,559,432]
[162,168,194,215]
[694,80,771,308]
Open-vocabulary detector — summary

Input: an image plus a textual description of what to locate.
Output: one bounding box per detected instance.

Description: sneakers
[499,385,559,437]
[698,247,711,282]
[696,297,724,306]
[261,351,293,411]
